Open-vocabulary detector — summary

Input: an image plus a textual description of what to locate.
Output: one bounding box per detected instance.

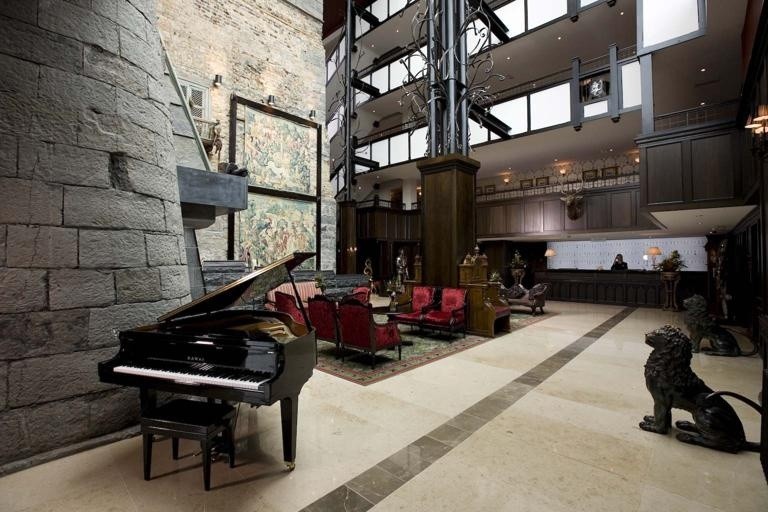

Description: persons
[612,253,628,271]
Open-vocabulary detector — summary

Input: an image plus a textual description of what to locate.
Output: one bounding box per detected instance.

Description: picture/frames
[227,92,322,271]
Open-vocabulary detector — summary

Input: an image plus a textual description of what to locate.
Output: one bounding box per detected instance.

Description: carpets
[314,308,561,387]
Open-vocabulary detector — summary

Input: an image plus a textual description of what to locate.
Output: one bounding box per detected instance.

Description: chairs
[275,285,470,370]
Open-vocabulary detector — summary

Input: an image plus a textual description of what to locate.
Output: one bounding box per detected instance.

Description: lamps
[745,103,768,155]
[544,248,557,269]
[647,247,662,270]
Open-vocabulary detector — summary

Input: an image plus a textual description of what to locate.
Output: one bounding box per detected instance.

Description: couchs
[263,279,323,309]
[495,282,551,317]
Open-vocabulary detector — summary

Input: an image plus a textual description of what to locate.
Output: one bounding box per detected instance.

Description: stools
[139,397,238,491]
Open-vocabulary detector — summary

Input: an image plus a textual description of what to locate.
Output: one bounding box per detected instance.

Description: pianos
[98,252,318,471]
